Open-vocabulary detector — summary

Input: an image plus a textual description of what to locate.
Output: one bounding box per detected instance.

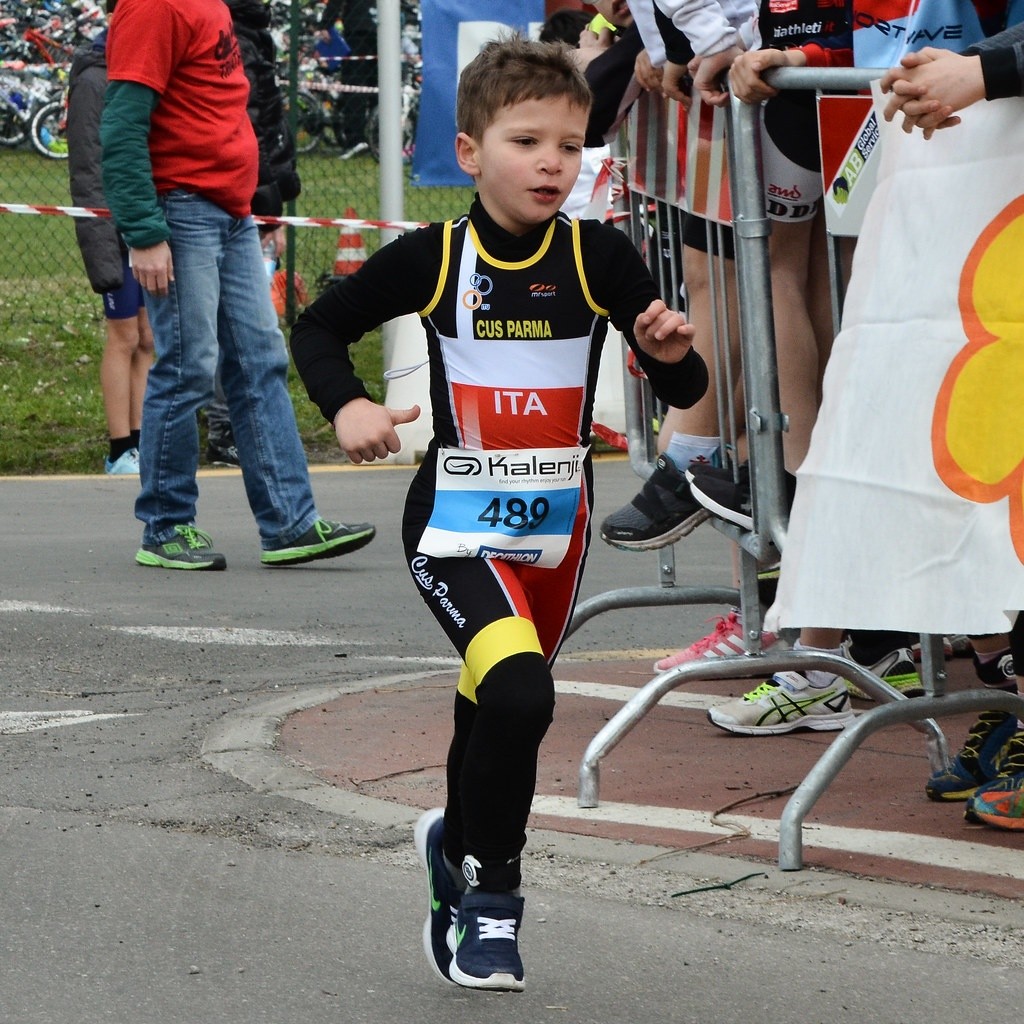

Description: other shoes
[948,636,973,655]
[971,649,1016,693]
[103,448,141,475]
[913,634,954,662]
[207,423,239,467]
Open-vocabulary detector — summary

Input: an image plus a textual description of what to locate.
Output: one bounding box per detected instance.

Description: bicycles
[0,0,421,166]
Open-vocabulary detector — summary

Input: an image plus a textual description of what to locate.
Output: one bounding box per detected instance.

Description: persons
[540,0,1024,829]
[66,0,155,475]
[99,0,377,570]
[289,41,710,994]
[206,0,301,469]
[320,0,377,159]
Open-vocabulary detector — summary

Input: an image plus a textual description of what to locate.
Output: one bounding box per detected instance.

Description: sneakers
[839,636,926,703]
[925,707,1016,800]
[598,453,709,551]
[135,524,227,570]
[685,459,749,493]
[260,514,376,565]
[414,804,458,987]
[654,611,786,673]
[969,784,1024,831]
[445,883,526,993]
[693,470,797,533]
[960,719,1024,824]
[707,664,857,734]
